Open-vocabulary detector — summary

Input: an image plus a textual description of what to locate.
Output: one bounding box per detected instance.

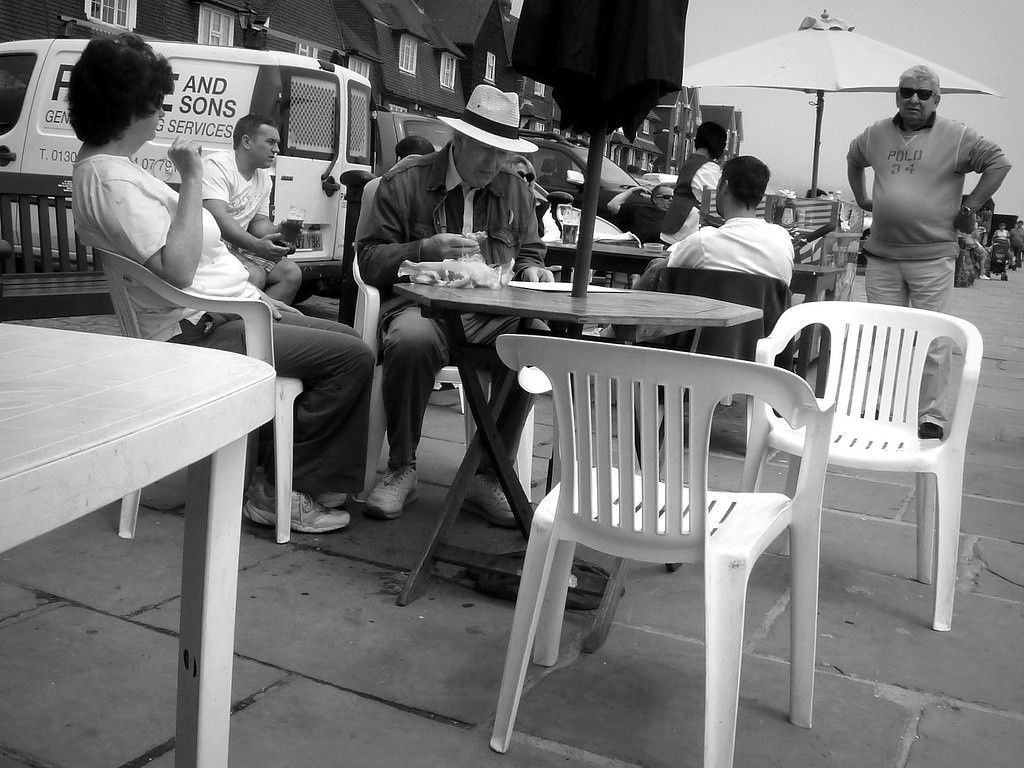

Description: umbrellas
[681,9,1004,198]
[512,0,691,299]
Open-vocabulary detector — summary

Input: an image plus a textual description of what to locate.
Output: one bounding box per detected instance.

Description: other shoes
[1016,263,1021,268]
[1012,265,1016,271]
[979,274,991,280]
[919,422,943,439]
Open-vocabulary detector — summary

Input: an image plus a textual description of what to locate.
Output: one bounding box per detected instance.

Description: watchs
[961,204,974,217]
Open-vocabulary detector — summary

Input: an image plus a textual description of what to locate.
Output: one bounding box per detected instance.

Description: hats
[436,83,540,154]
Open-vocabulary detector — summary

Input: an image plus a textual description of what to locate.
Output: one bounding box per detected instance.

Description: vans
[0,38,374,305]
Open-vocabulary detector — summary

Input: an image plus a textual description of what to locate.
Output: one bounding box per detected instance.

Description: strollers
[989,235,1012,282]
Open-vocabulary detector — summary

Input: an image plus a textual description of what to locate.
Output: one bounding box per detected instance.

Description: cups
[278,206,306,247]
[643,242,664,253]
[561,210,580,243]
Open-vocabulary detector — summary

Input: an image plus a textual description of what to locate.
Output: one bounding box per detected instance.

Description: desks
[0,317,279,768]
[539,241,847,406]
[392,277,765,655]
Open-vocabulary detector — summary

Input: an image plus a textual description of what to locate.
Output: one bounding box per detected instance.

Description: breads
[461,231,488,245]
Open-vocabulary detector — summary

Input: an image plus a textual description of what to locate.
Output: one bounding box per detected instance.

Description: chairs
[352,253,535,506]
[653,266,797,355]
[94,248,305,544]
[489,332,834,768]
[741,301,983,632]
[537,159,559,187]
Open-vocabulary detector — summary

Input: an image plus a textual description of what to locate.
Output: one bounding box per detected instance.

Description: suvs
[368,100,453,178]
[517,127,653,226]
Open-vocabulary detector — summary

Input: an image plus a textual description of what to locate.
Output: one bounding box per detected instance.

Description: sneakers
[313,481,348,510]
[362,457,419,520]
[242,466,351,534]
[461,468,520,529]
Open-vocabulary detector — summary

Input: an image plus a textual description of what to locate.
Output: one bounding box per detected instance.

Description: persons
[582,155,795,343]
[991,221,1011,247]
[508,152,561,243]
[958,211,991,279]
[352,135,436,254]
[199,111,304,306]
[846,64,1013,440]
[660,119,728,253]
[608,184,674,244]
[805,188,827,199]
[358,85,556,525]
[1009,220,1024,268]
[67,34,375,534]
[646,160,654,173]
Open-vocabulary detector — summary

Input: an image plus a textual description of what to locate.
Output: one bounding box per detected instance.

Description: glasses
[653,194,674,199]
[898,85,936,101]
